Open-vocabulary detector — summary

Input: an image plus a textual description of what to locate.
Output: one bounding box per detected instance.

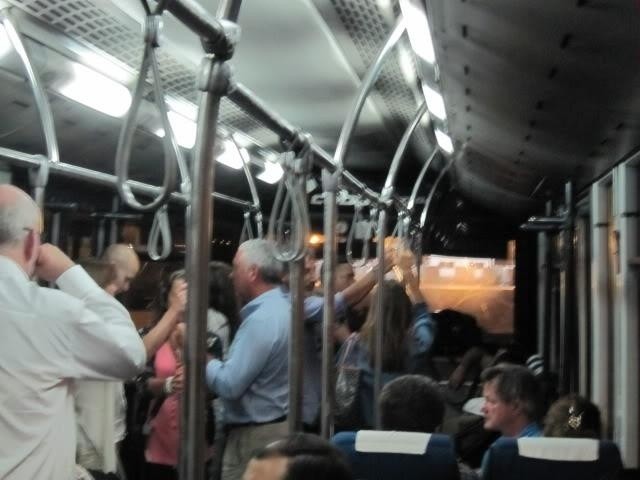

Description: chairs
[329,356,623,479]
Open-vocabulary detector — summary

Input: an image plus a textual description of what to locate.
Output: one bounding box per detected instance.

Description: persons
[0,182,148,480]
